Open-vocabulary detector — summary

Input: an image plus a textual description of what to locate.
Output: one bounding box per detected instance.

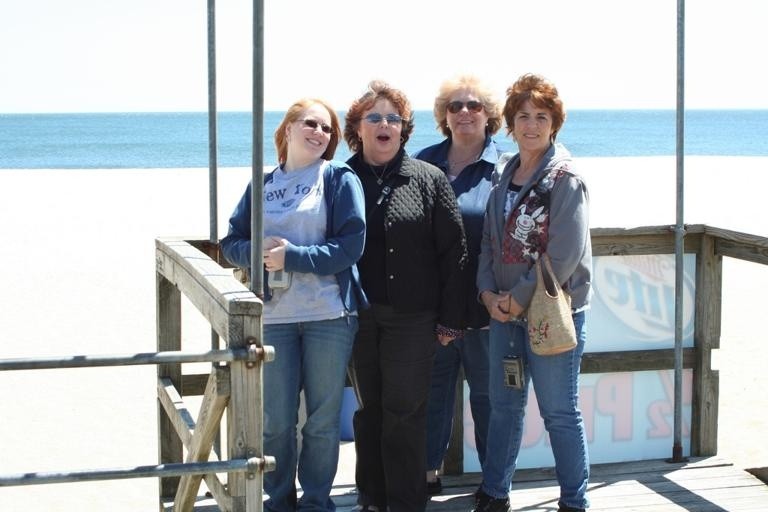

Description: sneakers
[426,477,443,495]
[472,482,512,512]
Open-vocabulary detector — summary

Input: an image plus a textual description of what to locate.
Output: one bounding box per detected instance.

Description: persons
[475,74,594,511]
[409,74,517,495]
[222,97,368,511]
[339,82,469,511]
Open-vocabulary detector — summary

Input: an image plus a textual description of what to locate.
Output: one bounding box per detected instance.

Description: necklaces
[447,144,484,171]
[363,157,395,185]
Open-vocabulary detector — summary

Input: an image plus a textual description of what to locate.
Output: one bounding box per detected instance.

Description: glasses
[297,118,332,134]
[362,113,403,125]
[445,101,484,113]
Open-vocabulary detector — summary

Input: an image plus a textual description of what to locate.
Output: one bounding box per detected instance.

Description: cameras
[503,353,525,388]
[267,268,291,290]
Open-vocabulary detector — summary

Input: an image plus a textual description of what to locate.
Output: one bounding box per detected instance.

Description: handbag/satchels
[527,255,578,357]
